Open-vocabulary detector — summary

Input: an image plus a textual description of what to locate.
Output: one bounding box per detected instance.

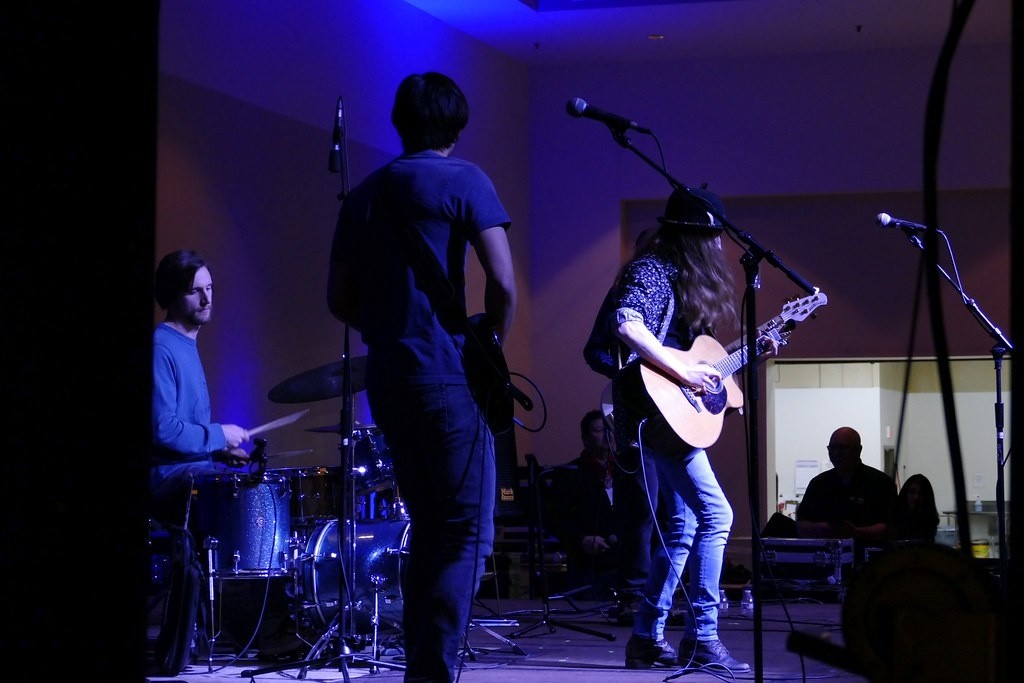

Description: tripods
[241,319,407,683]
[509,455,617,641]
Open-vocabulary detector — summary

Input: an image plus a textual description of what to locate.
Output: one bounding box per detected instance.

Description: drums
[171,471,293,580]
[301,516,411,635]
[264,464,343,526]
[338,430,396,494]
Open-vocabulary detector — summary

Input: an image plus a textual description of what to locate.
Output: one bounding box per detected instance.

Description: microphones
[875,213,944,235]
[328,100,344,174]
[566,98,654,135]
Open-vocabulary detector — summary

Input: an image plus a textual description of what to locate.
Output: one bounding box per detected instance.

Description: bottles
[975,496,982,511]
[740,589,753,615]
[719,589,731,617]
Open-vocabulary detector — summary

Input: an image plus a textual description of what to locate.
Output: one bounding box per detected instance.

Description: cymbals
[267,354,368,405]
[303,420,377,435]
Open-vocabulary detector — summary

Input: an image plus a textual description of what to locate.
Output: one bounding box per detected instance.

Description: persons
[583,184,787,675]
[149,249,250,491]
[757,427,897,558]
[328,71,516,683]
[840,543,1004,683]
[540,409,670,584]
[896,474,939,543]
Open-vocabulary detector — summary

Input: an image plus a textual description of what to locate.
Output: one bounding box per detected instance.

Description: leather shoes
[679,638,751,674]
[624,633,681,669]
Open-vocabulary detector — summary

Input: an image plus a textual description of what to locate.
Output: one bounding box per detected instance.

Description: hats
[657,187,726,233]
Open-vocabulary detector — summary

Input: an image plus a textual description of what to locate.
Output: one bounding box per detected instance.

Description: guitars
[611,291,828,464]
[458,313,514,438]
